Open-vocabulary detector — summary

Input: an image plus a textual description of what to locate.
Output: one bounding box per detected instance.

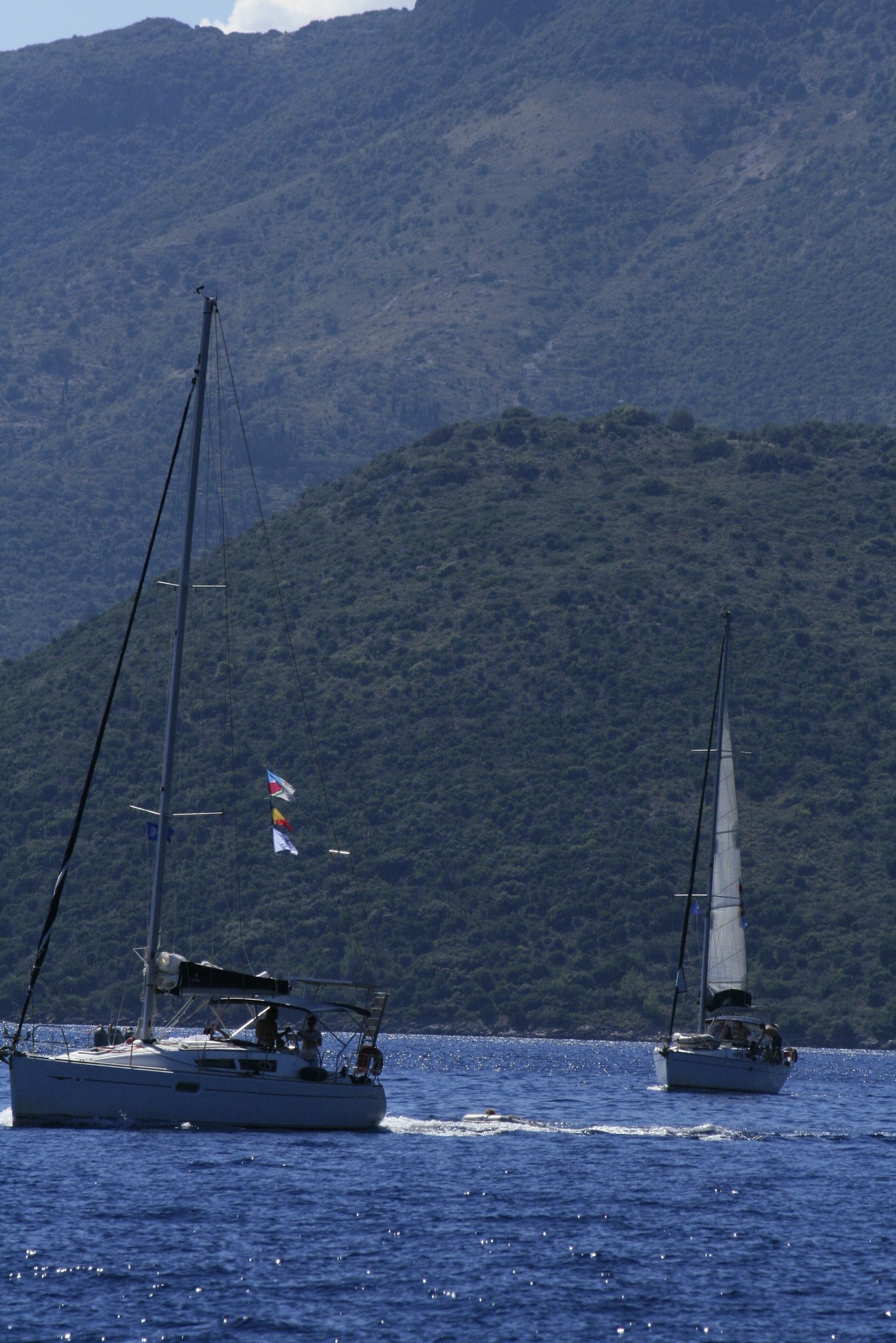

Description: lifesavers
[356,1046,383,1076]
[783,1048,798,1063]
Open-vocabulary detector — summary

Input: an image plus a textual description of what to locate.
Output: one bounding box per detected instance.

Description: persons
[296,1016,322,1068]
[757,1023,783,1064]
[255,1007,291,1047]
[713,1021,774,1063]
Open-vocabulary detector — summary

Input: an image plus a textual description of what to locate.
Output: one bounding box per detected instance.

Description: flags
[147,821,175,843]
[691,900,702,915]
[739,879,744,895]
[266,769,297,802]
[740,900,745,916]
[272,828,299,857]
[270,802,293,832]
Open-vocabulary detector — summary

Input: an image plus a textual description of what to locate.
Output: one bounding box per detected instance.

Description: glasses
[307,1022,313,1025]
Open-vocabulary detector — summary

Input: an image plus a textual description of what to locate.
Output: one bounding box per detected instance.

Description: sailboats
[651,601,800,1097]
[0,264,392,1137]
